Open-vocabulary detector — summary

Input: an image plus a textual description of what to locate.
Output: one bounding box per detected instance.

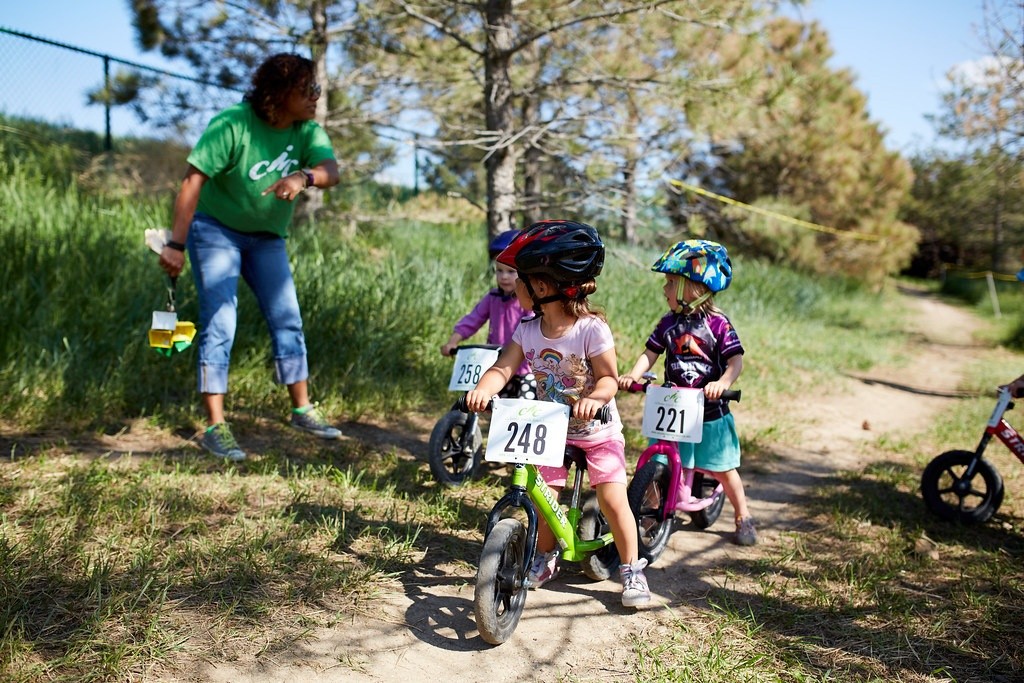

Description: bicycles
[619,372,742,564]
[458,394,620,646]
[428,344,504,493]
[920,385,1023,536]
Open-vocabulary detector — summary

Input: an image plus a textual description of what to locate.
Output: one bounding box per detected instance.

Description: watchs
[300,167,315,190]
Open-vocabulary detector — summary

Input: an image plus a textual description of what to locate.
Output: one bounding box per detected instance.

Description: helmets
[651,239,732,292]
[496,220,606,282]
[488,230,522,258]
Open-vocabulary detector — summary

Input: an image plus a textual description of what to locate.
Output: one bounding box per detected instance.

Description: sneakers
[641,518,658,545]
[198,423,246,461]
[735,516,756,546]
[620,564,651,607]
[527,547,562,589]
[290,401,342,438]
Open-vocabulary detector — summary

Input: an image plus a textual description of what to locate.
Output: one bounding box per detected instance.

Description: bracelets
[167,238,187,252]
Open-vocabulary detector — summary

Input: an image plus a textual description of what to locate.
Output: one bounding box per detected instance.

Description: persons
[444,230,528,406]
[996,267,1024,402]
[466,219,649,608]
[158,52,343,460]
[616,241,756,548]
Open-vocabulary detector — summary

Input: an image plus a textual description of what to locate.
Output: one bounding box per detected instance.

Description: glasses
[293,83,321,96]
